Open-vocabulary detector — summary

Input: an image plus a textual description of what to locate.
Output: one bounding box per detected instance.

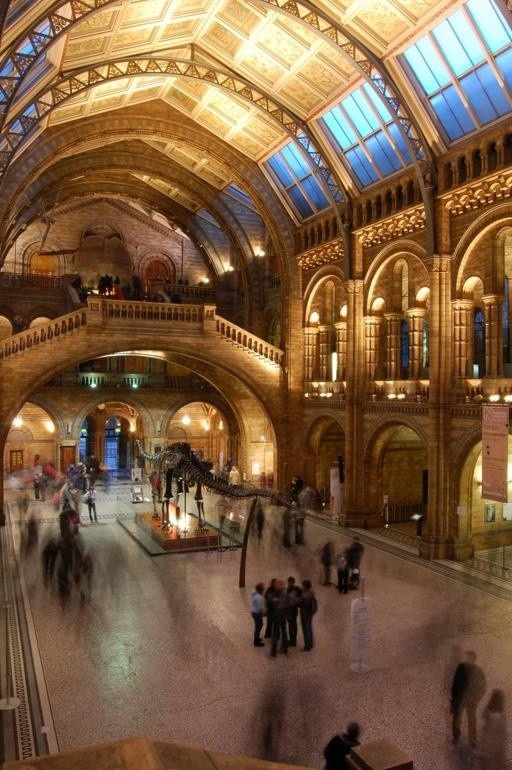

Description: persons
[97,274,189,304]
[323,723,362,769]
[449,650,486,748]
[482,690,507,769]
